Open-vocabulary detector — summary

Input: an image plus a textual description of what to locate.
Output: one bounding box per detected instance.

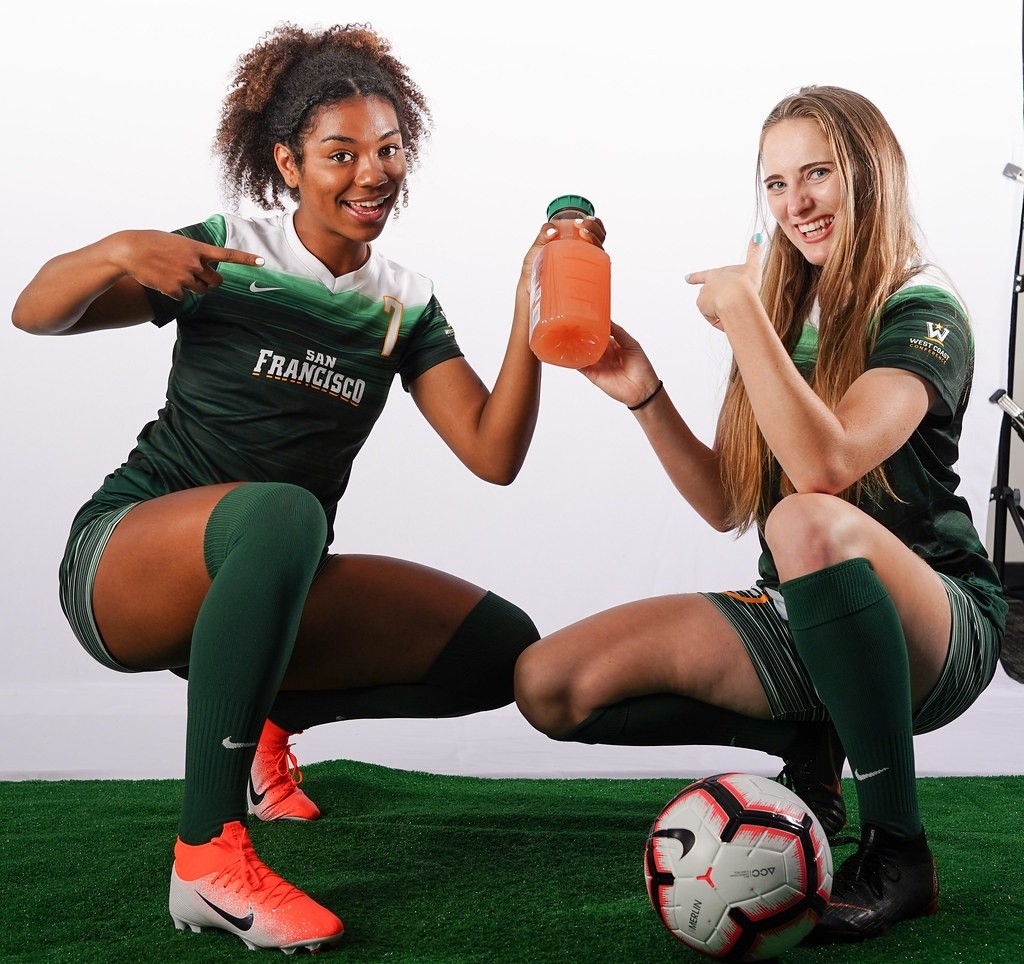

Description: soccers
[644,771,833,963]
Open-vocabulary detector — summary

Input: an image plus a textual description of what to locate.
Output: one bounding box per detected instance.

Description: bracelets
[626,381,664,410]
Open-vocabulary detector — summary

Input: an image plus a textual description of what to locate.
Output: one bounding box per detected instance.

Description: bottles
[526,194,612,369]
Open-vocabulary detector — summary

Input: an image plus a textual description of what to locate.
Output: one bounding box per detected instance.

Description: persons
[514,88,1008,949]
[10,19,605,955]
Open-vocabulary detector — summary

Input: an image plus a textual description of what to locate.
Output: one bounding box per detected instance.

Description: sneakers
[777,742,847,842]
[815,823,940,934]
[248,717,321,822]
[167,821,346,954]
